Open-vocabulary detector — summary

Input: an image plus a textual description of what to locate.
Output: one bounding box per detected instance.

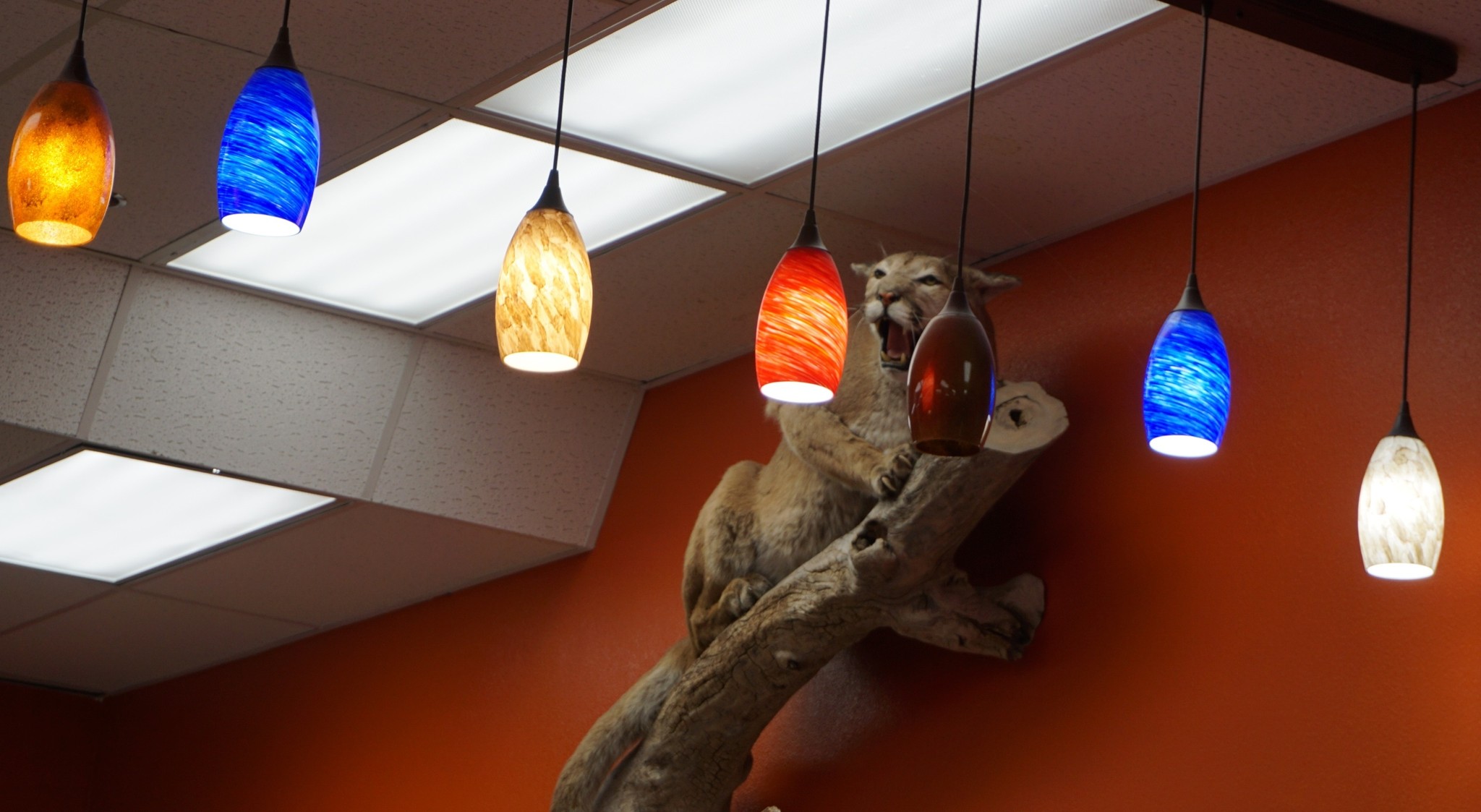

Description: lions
[684,251,1013,663]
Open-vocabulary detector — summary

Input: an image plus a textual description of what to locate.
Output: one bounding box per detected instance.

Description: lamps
[1358,58,1444,581]
[216,0,321,239]
[907,0,995,459]
[1143,0,1232,460]
[755,0,849,404]
[495,0,593,374]
[7,0,115,250]
